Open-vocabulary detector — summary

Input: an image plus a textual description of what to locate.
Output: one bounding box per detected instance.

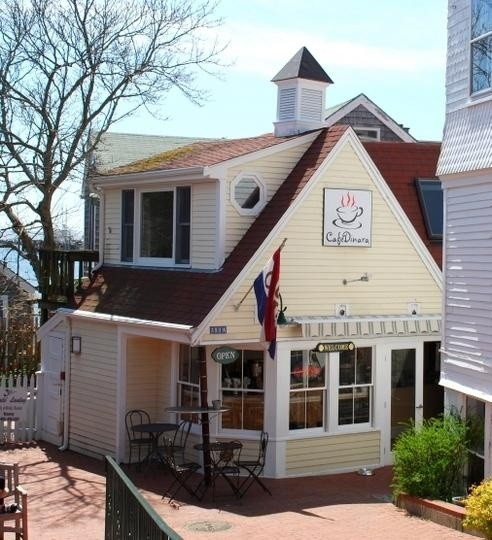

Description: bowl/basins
[358,468,375,475]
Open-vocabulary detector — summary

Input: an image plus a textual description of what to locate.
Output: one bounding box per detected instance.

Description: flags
[253,242,283,360]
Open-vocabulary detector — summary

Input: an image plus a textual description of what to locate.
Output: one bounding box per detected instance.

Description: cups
[212,399,221,410]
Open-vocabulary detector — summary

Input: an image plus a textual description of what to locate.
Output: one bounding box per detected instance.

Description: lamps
[70,336,81,353]
[274,292,288,327]
[343,276,370,285]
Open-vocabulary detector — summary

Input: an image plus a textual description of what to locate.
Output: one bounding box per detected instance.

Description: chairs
[124,409,272,506]
[0,460,28,540]
